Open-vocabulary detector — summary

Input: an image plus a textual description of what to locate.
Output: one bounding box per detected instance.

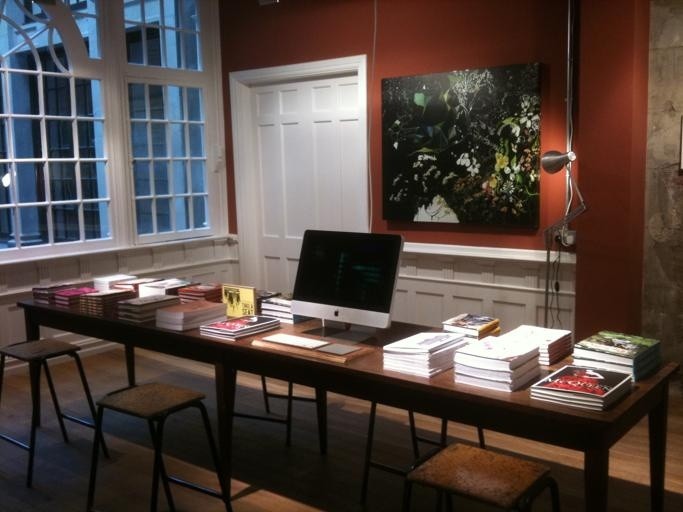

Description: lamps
[540,149,586,247]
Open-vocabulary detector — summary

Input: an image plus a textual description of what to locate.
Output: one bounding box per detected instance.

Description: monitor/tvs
[290,230,405,344]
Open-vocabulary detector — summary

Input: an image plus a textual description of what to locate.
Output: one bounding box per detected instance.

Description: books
[383,313,661,412]
[33,274,314,341]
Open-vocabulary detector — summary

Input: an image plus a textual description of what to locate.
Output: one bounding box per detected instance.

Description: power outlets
[552,230,577,247]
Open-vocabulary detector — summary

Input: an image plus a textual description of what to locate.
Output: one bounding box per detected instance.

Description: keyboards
[262,333,330,349]
